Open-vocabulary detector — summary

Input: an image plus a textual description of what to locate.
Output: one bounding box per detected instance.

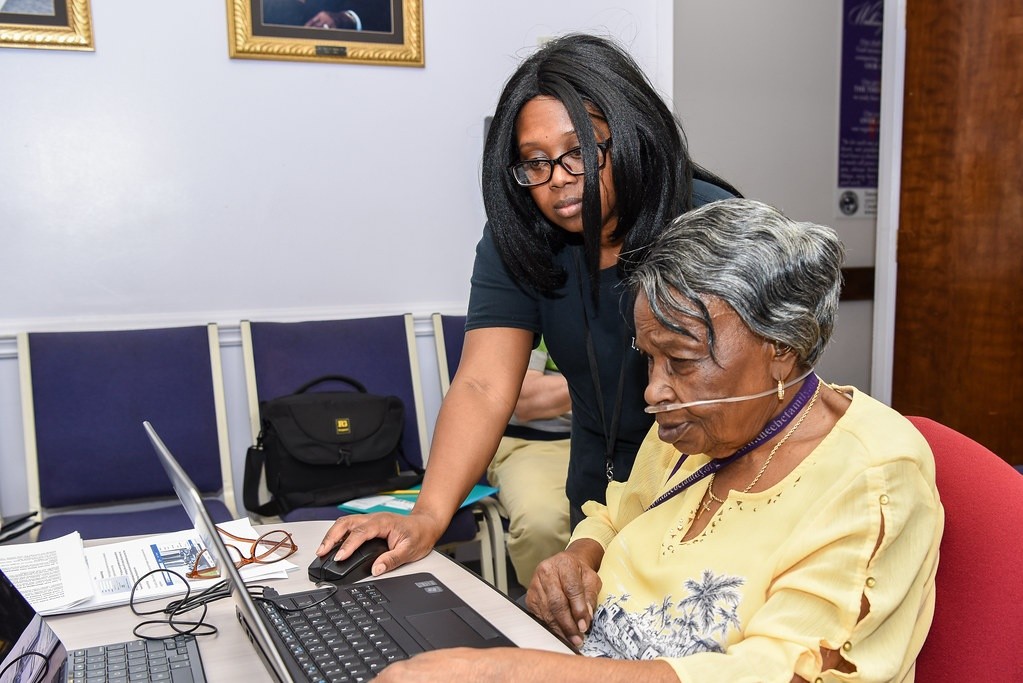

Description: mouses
[308,537,390,585]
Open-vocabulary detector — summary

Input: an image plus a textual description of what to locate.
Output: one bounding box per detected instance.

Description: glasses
[187,523,298,579]
[506,136,613,187]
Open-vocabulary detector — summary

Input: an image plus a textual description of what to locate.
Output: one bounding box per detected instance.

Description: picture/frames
[227,0,426,68]
[0,0,94,52]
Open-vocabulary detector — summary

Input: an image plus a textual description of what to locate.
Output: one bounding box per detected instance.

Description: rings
[323,24,329,29]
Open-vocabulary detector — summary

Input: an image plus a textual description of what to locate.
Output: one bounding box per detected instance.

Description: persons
[486,334,572,593]
[263,0,392,32]
[317,31,744,593]
[361,197,947,683]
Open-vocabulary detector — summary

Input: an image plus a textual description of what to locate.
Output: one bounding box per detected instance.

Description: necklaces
[696,377,823,517]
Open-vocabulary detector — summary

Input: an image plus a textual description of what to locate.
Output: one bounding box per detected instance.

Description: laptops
[143,421,518,683]
[0,568,208,683]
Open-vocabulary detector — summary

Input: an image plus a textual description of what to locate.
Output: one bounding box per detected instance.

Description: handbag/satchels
[243,375,428,518]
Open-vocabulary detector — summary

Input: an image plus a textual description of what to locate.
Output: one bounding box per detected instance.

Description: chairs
[435,309,587,599]
[900,411,1023,683]
[238,313,494,594]
[15,322,240,543]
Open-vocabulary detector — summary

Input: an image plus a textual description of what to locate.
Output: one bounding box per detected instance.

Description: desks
[44,519,583,683]
